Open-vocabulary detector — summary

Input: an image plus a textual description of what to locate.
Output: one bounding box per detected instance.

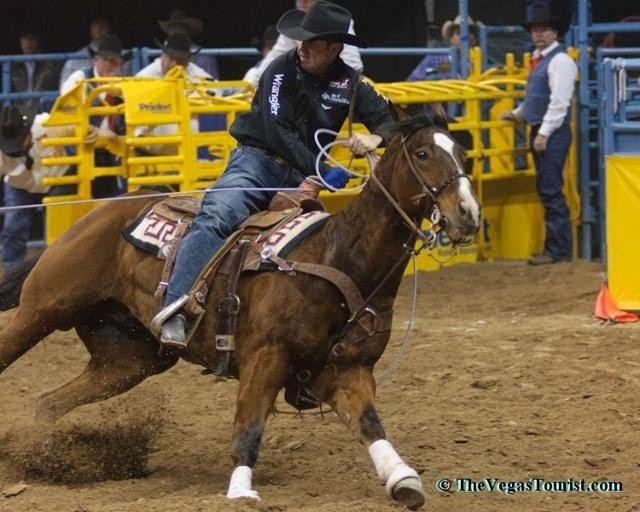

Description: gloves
[323,167,350,193]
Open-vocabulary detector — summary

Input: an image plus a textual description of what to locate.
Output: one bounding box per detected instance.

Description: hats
[0,101,39,154]
[87,2,571,63]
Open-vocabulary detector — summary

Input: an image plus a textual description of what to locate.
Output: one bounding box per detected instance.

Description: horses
[0,100,481,512]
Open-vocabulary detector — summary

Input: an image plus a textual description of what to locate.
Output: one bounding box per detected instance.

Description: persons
[0,0,364,271]
[406,14,475,122]
[160,2,400,347]
[501,4,577,266]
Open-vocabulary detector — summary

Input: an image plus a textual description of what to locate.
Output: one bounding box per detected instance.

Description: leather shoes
[159,313,188,350]
[530,252,574,265]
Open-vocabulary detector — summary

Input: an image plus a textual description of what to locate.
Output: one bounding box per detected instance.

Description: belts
[241,145,301,173]
